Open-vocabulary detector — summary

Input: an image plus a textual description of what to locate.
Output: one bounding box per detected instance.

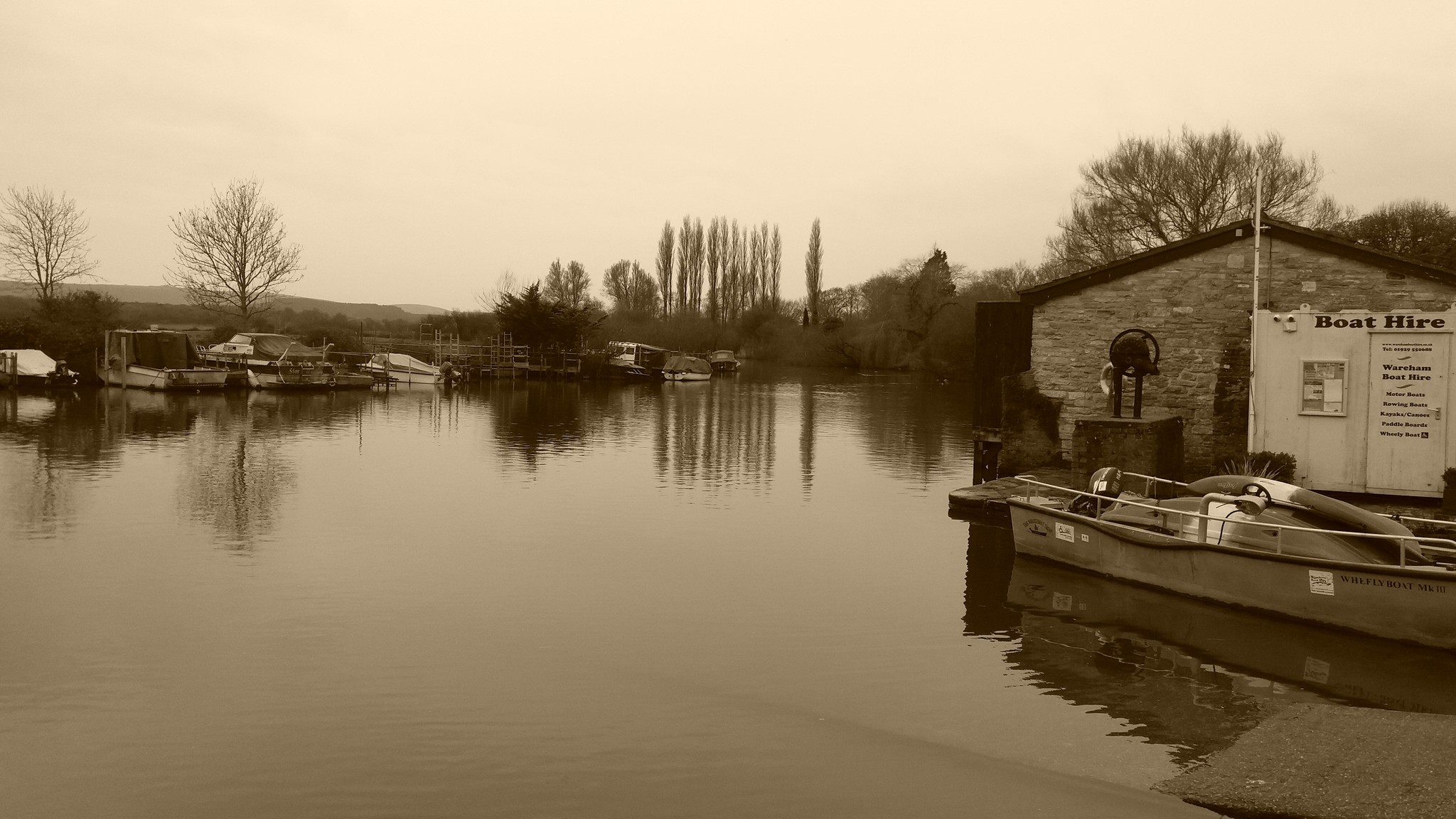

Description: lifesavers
[1101,362,1128,398]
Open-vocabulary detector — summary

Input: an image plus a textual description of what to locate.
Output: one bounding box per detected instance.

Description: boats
[710,350,737,371]
[661,356,713,381]
[95,329,228,392]
[1006,465,1456,649]
[0,349,80,388]
[625,371,650,382]
[195,332,374,390]
[355,352,461,385]
